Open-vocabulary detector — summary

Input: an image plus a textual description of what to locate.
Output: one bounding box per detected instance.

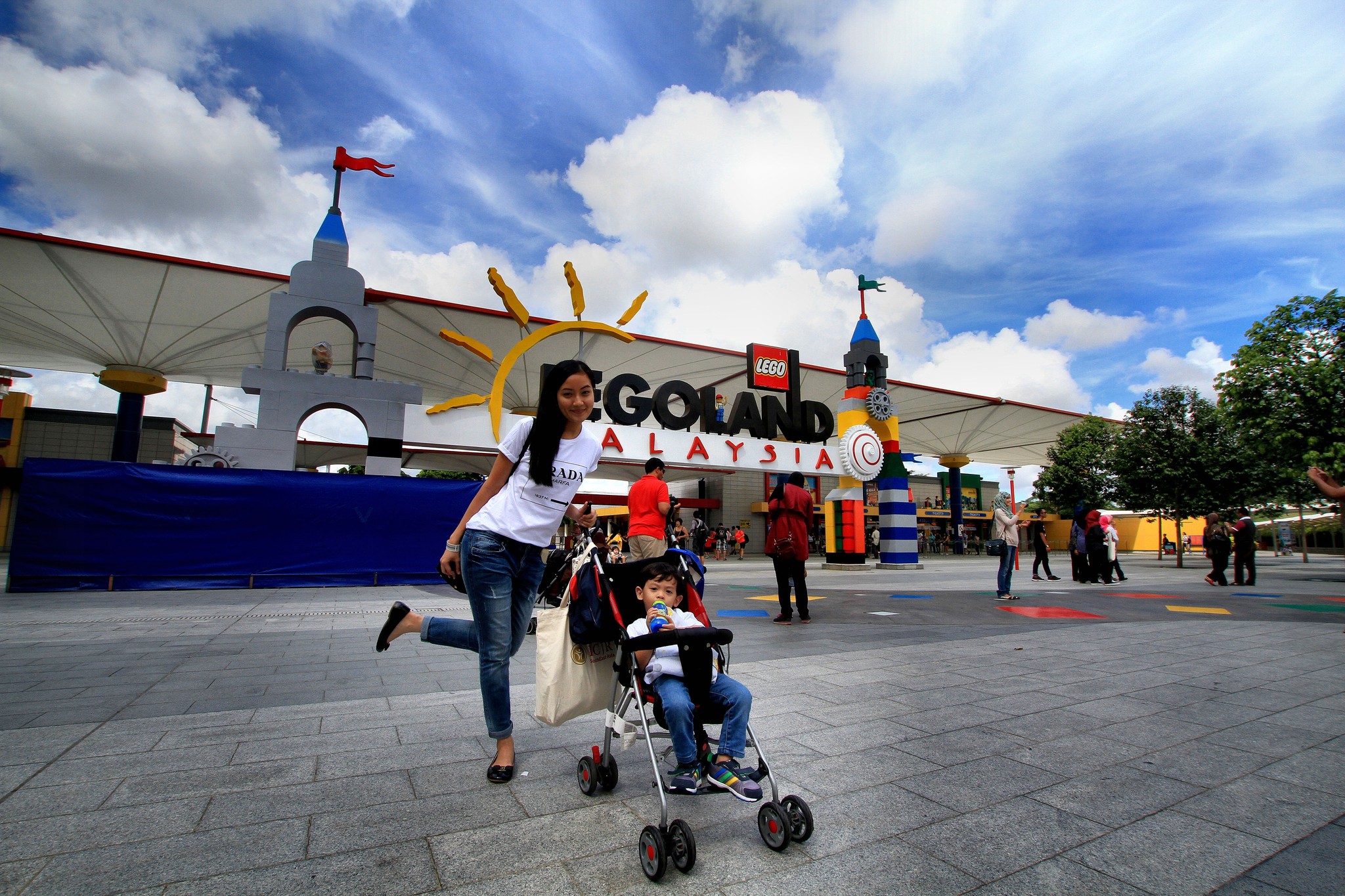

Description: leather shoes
[487,750,516,783]
[376,601,410,653]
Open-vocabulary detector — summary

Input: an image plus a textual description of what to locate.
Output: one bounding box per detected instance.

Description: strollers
[576,526,815,887]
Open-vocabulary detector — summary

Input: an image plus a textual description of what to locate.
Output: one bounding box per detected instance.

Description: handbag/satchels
[985,540,1009,557]
[438,555,468,594]
[735,540,740,549]
[1107,533,1116,563]
[675,537,678,543]
[744,533,749,544]
[1213,533,1230,551]
[774,538,796,561]
[534,543,622,727]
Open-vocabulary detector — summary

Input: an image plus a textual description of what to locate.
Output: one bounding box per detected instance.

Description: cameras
[669,496,679,507]
[1021,502,1029,508]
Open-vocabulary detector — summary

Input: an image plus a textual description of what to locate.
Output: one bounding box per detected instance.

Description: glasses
[660,467,665,474]
[1041,512,1046,514]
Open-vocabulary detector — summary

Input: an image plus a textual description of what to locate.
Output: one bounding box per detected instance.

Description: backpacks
[1184,536,1191,544]
[693,519,708,541]
[718,527,725,537]
[569,549,621,645]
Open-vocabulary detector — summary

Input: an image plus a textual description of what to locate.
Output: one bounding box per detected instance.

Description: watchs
[1046,545,1049,547]
[1018,524,1023,528]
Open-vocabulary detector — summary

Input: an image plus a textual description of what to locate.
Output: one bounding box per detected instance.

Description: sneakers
[707,753,763,802]
[800,616,811,623]
[773,614,791,624]
[668,760,701,793]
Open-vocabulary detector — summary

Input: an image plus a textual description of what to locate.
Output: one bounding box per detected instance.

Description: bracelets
[1226,526,1229,529]
[445,539,460,552]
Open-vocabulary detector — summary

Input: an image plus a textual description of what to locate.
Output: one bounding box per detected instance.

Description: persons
[1307,466,1345,633]
[993,491,1031,600]
[570,458,880,626]
[715,395,727,422]
[917,531,980,555]
[614,563,763,802]
[1162,534,1170,555]
[1068,508,1128,585]
[1201,508,1255,586]
[990,501,994,512]
[1032,508,1061,581]
[1181,532,1193,555]
[925,496,945,510]
[375,360,598,785]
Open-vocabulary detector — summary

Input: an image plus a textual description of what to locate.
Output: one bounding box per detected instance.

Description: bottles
[649,600,670,633]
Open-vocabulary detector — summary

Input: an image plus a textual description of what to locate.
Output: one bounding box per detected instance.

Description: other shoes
[1000,575,1128,600]
[872,557,879,559]
[704,549,743,560]
[1204,576,1255,586]
[918,550,979,555]
